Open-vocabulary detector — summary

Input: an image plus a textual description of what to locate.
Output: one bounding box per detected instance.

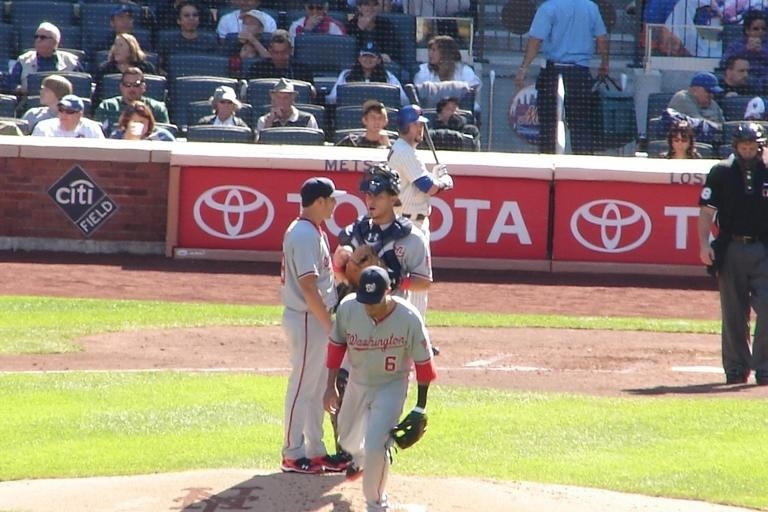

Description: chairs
[0,1,486,156]
[595,1,767,167]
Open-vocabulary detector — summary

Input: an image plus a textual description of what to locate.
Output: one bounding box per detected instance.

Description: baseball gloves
[390,411,428,450]
[345,246,388,290]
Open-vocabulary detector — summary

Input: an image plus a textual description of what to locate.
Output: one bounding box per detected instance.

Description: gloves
[432,165,453,191]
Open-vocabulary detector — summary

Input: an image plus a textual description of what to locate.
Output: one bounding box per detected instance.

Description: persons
[386,104,454,355]
[696,124,767,386]
[323,265,437,512]
[0,1,482,153]
[333,164,435,483]
[515,0,610,152]
[279,177,349,474]
[631,2,768,157]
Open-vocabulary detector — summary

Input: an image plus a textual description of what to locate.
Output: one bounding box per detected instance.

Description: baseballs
[341,244,352,255]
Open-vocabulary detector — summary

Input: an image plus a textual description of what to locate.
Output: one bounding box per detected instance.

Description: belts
[733,233,758,244]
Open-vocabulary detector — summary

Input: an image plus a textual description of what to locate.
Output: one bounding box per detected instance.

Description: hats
[270,78,298,94]
[690,71,730,93]
[359,42,378,57]
[238,10,266,29]
[357,266,391,305]
[401,104,428,125]
[214,86,236,102]
[744,96,767,119]
[109,5,128,16]
[57,95,84,113]
[300,177,346,206]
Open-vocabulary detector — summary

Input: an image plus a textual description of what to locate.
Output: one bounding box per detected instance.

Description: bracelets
[332,263,346,273]
[401,274,410,291]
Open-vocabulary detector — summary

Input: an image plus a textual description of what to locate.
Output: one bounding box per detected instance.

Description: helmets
[732,122,763,169]
[358,166,398,195]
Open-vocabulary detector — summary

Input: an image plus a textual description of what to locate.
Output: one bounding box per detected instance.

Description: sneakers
[281,455,363,480]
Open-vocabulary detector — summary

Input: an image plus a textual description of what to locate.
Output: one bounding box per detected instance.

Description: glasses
[34,34,51,40]
[59,107,74,114]
[121,80,141,88]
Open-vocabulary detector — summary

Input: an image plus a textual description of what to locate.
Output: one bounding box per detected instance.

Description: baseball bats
[406,83,454,190]
